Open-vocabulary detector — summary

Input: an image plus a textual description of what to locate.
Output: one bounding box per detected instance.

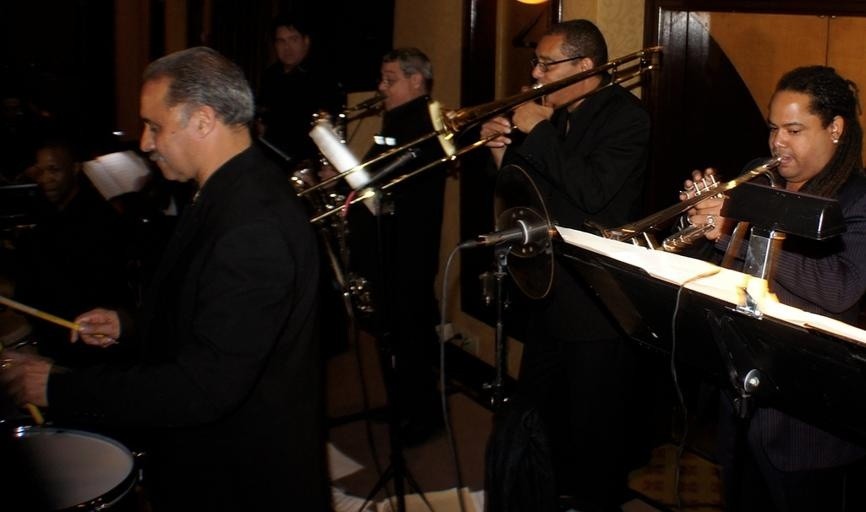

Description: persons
[480,18,657,510]
[315,44,450,451]
[1,137,128,355]
[1,43,341,512]
[250,8,340,164]
[677,63,866,509]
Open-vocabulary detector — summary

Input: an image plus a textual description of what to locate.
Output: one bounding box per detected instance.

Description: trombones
[295,46,662,225]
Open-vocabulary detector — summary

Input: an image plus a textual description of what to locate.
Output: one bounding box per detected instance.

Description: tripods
[356,296,433,512]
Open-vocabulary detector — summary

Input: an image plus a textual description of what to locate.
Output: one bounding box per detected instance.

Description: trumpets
[578,152,782,255]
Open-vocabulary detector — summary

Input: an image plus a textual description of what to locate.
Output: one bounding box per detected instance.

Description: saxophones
[291,93,386,319]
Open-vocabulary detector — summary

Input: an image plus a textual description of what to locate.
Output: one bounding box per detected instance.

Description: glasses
[528,55,586,73]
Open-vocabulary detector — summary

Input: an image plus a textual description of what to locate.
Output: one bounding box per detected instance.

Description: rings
[705,215,717,225]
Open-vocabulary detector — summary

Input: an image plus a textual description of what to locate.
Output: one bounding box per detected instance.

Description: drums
[0,312,38,355]
[0,426,151,512]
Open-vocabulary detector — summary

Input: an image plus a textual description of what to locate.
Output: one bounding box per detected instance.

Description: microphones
[481,217,558,249]
